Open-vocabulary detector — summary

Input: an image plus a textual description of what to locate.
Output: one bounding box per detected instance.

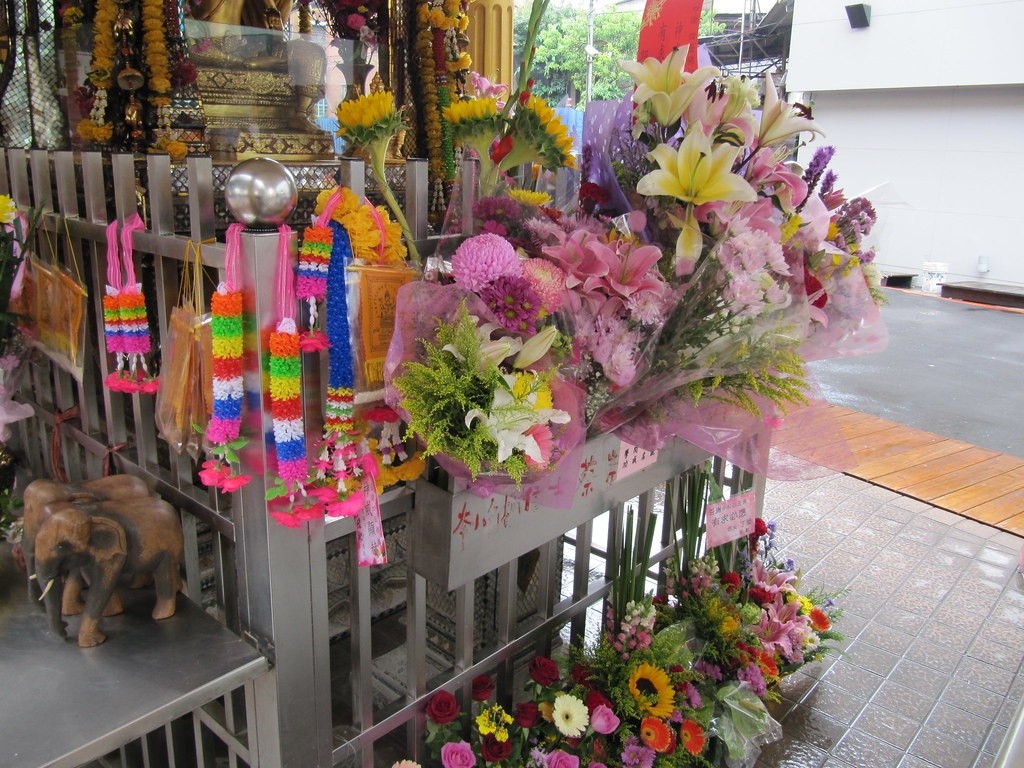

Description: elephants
[22,474,160,616]
[29,498,184,647]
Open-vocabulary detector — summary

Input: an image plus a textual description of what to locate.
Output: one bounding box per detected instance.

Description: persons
[183,0,331,135]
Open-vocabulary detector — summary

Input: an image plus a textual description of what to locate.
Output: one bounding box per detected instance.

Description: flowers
[0,194,51,442]
[334,45,894,768]
